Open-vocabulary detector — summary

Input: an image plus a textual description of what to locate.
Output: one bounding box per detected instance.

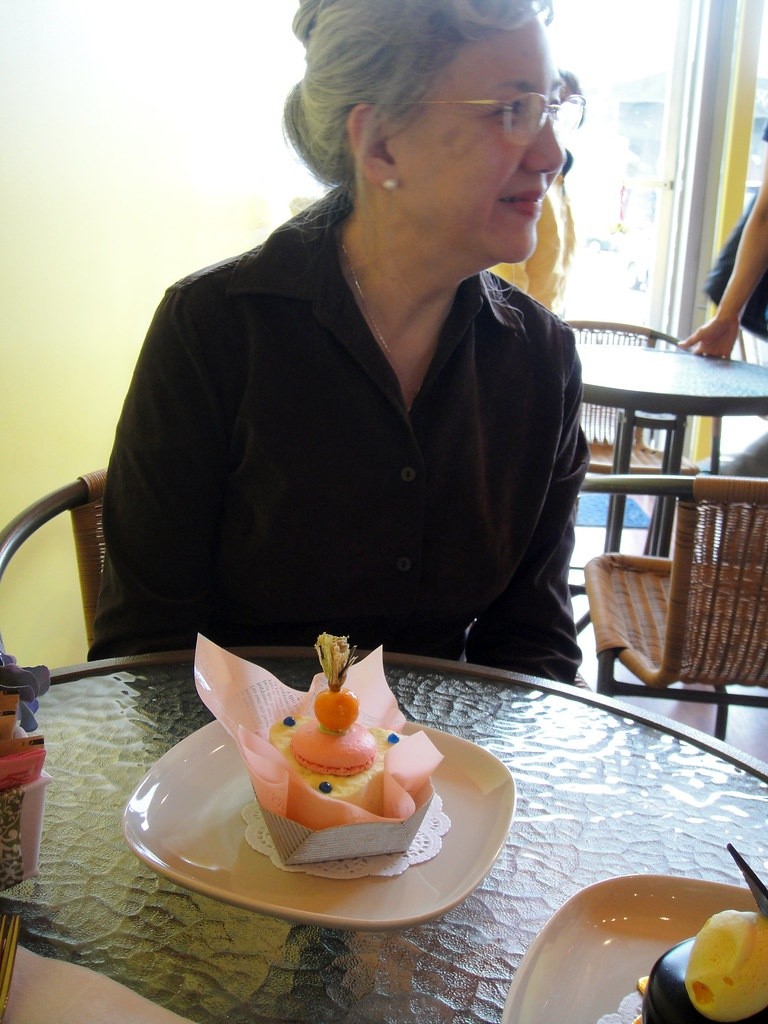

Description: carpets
[572,492,653,529]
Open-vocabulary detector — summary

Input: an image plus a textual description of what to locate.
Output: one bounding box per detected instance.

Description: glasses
[370,93,586,145]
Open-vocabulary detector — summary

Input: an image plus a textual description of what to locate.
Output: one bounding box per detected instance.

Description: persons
[678,125,768,477]
[491,57,581,316]
[84,1,586,688]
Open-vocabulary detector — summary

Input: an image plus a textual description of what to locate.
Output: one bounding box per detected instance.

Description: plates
[123,719,517,927]
[501,871,759,1024]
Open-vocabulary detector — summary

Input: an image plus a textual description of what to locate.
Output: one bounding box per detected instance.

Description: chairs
[584,473,768,742]
[0,471,115,674]
[562,319,699,558]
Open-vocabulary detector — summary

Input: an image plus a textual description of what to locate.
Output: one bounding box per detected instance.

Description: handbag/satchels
[706,193,768,341]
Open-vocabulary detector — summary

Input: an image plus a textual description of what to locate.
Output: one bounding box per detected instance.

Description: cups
[0,769,54,892]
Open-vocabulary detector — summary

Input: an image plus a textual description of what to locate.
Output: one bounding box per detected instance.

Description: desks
[0,644,768,1024]
[569,346,768,636]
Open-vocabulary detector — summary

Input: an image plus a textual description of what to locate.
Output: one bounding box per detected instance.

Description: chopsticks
[0,914,20,1022]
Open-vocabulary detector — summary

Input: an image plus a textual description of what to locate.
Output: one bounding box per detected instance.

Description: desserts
[642,842,768,1024]
[268,634,404,816]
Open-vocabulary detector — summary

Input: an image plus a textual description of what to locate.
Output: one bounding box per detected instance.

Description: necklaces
[335,218,425,398]
